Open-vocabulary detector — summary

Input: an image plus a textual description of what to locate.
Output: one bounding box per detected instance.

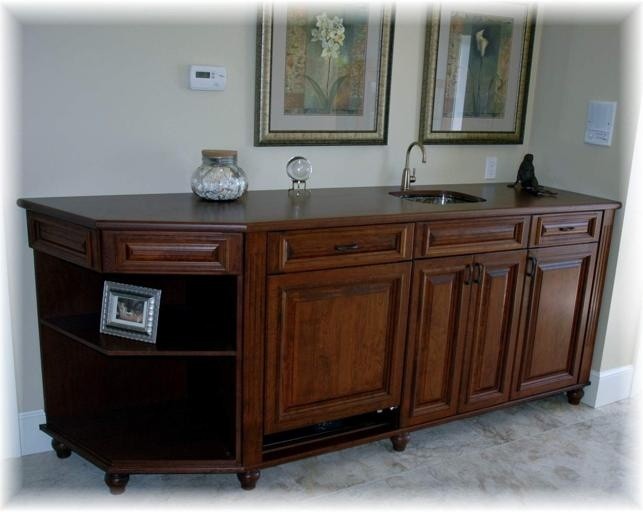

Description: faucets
[401,141,426,191]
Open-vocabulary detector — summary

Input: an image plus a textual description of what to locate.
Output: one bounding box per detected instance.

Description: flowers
[295,12,354,114]
[464,29,497,116]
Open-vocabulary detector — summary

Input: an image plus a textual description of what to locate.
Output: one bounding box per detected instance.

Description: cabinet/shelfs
[400,217,529,444]
[242,222,417,493]
[508,208,615,406]
[27,210,245,498]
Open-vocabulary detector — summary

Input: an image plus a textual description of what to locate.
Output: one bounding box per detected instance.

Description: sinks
[388,190,486,204]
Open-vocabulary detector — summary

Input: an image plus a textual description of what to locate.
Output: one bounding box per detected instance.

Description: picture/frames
[418,6,537,147]
[255,5,397,147]
[99,278,165,346]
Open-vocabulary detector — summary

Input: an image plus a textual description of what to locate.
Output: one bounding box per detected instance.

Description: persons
[507,153,558,198]
